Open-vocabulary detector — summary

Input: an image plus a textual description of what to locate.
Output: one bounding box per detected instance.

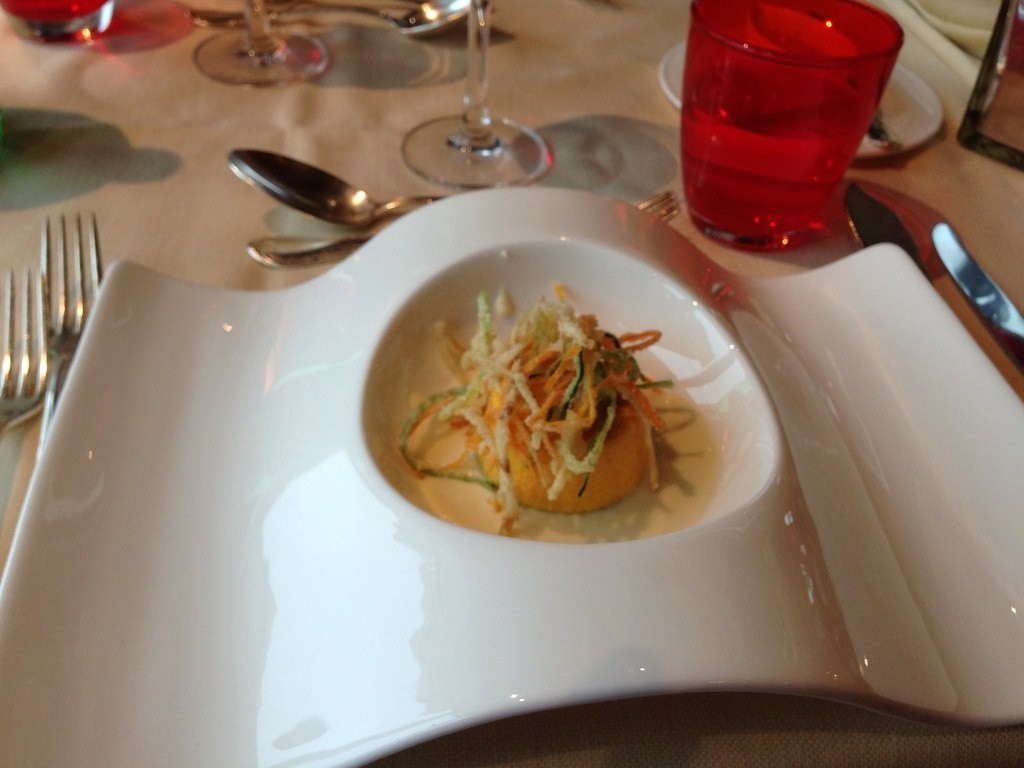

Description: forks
[0,269,45,438]
[39,213,103,443]
[248,190,681,266]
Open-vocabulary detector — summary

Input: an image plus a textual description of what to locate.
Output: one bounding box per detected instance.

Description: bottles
[958,0,1024,173]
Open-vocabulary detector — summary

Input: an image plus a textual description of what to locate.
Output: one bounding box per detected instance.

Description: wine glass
[191,1,329,86]
[402,2,551,190]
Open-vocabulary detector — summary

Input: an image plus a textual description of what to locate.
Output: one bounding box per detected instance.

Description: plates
[659,41,945,155]
[0,186,1024,768]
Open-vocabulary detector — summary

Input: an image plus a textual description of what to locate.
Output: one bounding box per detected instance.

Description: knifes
[933,224,1023,378]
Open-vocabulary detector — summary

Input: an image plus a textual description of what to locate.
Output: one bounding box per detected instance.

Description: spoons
[187,0,487,34]
[225,148,445,227]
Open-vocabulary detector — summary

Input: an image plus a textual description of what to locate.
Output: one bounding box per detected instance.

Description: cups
[0,1,116,45]
[679,1,906,249]
